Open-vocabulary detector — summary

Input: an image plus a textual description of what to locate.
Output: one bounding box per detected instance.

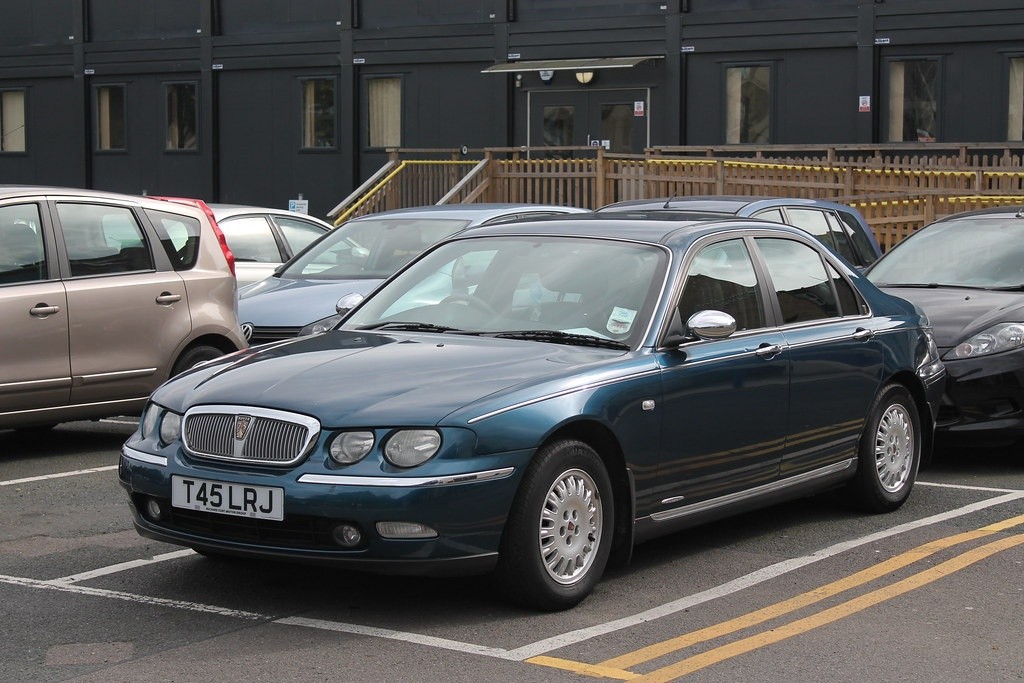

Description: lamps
[575,71,599,87]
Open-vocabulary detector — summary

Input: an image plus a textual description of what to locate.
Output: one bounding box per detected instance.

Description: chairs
[559,255,683,344]
[381,226,421,270]
[516,256,589,324]
[3,223,47,282]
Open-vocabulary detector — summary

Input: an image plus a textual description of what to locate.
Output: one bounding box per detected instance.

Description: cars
[590,194,883,276]
[858,204,1024,449]
[101,203,371,294]
[236,203,594,349]
[117,207,948,610]
[1,182,250,441]
